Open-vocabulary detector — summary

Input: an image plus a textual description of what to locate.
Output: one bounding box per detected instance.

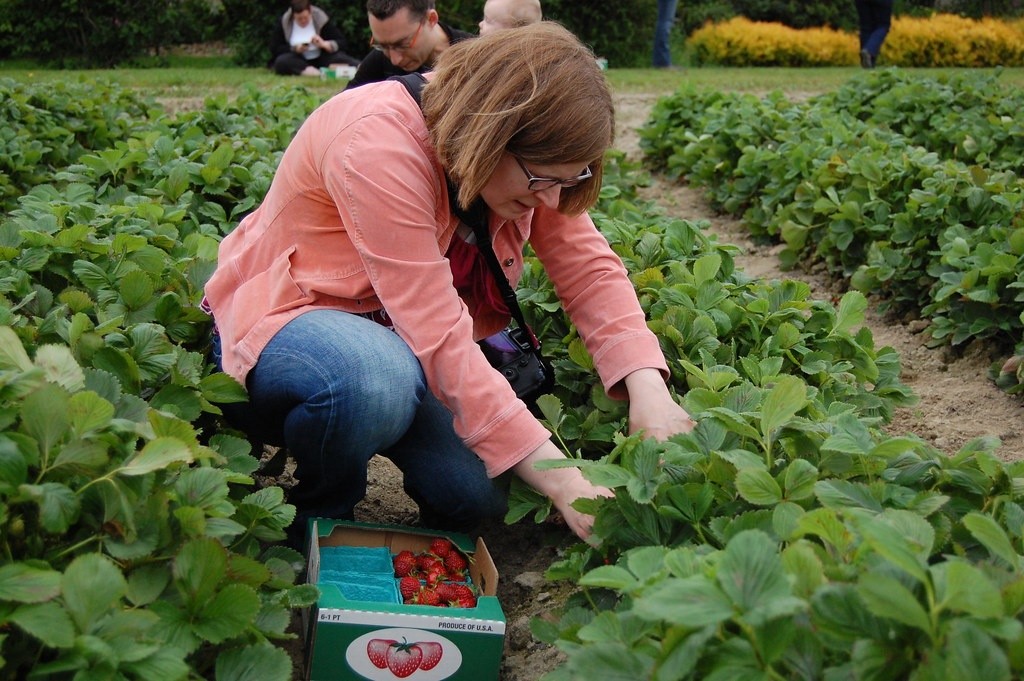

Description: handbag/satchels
[475,325,555,416]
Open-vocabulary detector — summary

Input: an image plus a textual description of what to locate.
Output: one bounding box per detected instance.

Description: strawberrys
[394,540,476,609]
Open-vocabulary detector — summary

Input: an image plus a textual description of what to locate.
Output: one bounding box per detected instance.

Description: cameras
[476,326,556,407]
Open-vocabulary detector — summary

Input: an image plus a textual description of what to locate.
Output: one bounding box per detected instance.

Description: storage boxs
[319,66,358,82]
[300,518,507,681]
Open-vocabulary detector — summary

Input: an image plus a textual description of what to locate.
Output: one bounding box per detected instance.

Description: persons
[274,0,893,92]
[199,21,698,551]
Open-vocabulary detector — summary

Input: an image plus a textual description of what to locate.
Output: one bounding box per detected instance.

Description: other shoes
[860,49,872,69]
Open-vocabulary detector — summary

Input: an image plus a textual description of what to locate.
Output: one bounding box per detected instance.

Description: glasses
[507,142,592,192]
[370,15,426,51]
